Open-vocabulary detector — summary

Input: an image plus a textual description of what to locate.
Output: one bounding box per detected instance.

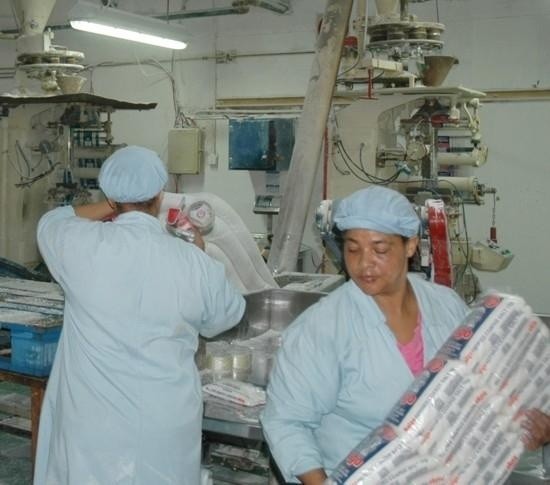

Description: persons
[259,183,548,483]
[31,145,246,485]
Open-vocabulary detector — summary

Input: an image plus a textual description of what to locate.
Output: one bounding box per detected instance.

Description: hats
[99,145,168,203]
[333,184,422,238]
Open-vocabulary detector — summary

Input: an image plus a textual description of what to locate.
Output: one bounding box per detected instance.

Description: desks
[0,369,49,482]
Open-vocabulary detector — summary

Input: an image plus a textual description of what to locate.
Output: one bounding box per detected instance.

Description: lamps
[68,0,190,49]
